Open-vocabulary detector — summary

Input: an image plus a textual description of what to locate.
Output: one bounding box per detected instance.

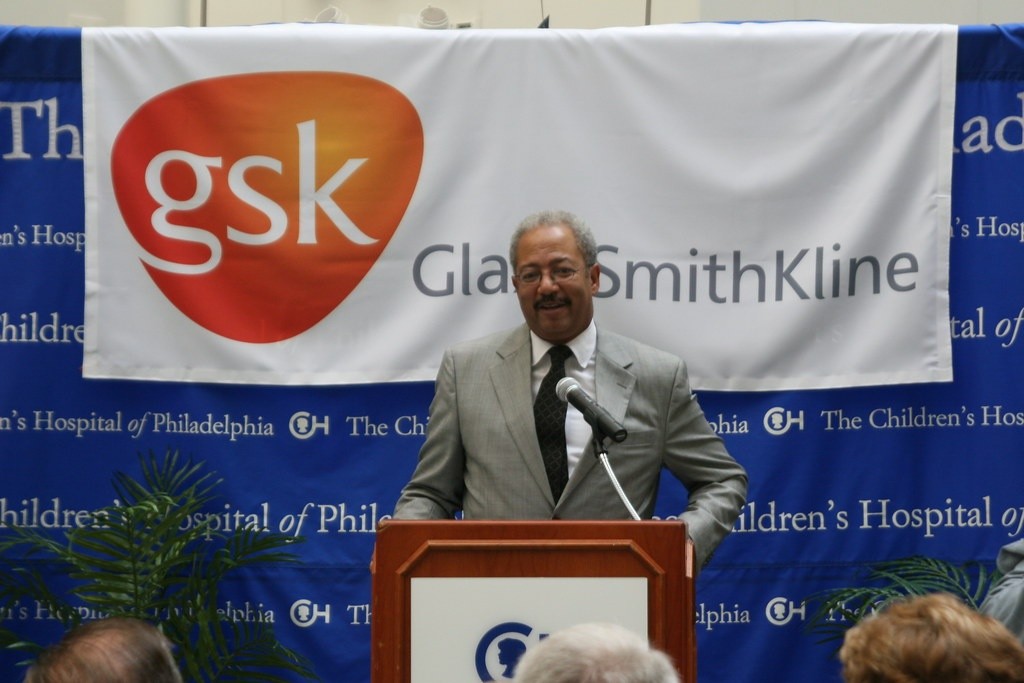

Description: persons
[511,623,679,683]
[838,592,1024,683]
[23,617,182,683]
[391,209,749,573]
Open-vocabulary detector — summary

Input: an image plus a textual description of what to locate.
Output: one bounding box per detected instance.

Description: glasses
[515,265,590,286]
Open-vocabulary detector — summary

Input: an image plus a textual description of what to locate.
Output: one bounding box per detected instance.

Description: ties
[534,346,573,507]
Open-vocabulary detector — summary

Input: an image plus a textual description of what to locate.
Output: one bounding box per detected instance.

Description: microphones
[556,377,627,443]
